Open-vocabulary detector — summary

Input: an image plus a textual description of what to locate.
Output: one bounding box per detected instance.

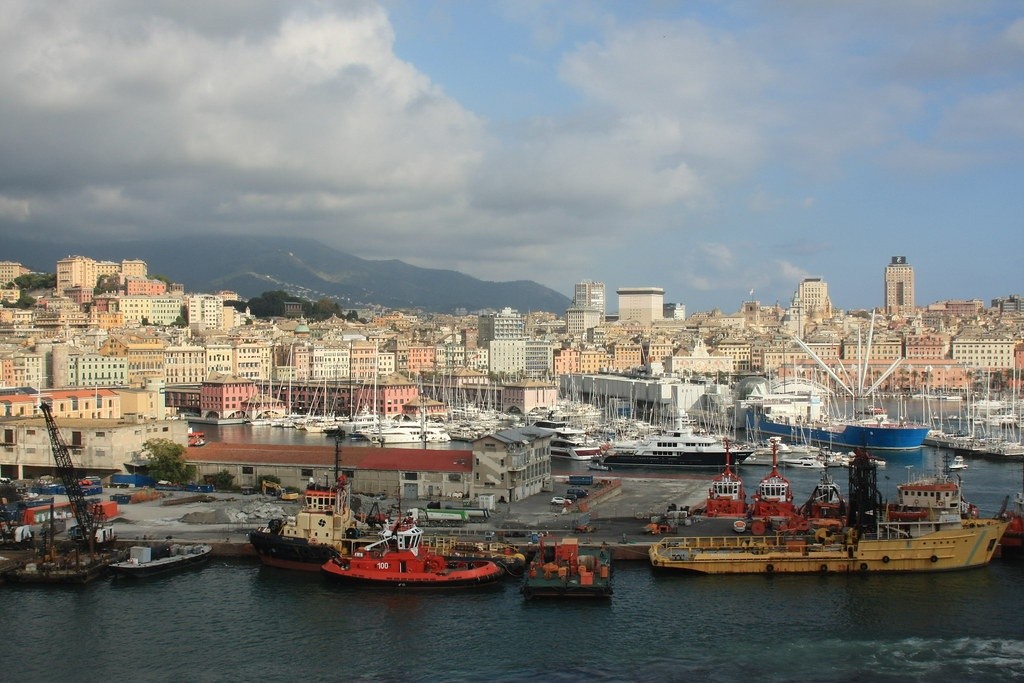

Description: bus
[569,476,594,485]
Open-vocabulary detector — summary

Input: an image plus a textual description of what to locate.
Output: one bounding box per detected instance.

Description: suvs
[551,497,572,504]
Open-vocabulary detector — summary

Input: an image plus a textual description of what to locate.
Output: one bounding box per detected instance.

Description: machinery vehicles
[39,403,106,552]
[262,480,299,504]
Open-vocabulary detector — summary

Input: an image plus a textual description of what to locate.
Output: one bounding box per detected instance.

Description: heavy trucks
[405,506,469,527]
[428,500,492,523]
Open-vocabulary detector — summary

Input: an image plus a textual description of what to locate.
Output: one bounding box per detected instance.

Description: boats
[250,339,528,444]
[743,401,933,450]
[188,428,206,447]
[859,357,1024,459]
[529,537,614,595]
[245,430,524,585]
[109,544,214,577]
[648,436,1024,573]
[528,379,754,471]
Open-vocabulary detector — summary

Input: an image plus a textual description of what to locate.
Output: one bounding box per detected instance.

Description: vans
[567,488,588,498]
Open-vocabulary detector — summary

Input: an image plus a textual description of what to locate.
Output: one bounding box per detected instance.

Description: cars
[565,494,578,501]
